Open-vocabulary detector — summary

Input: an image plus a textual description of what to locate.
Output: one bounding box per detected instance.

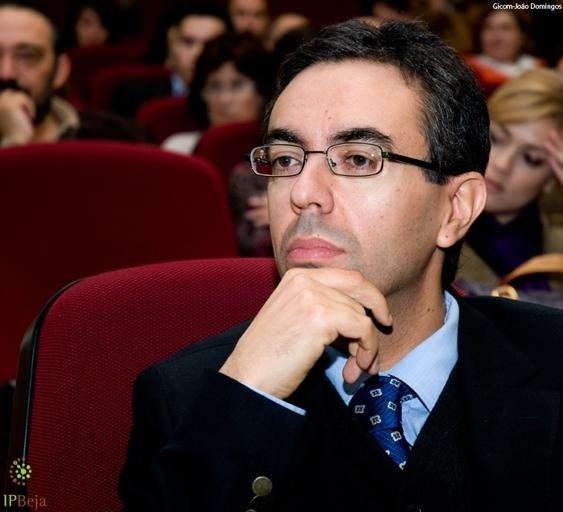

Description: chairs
[1,39,273,512]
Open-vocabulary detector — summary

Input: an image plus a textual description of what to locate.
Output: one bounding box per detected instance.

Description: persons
[116,17,563,512]
[0,0,563,288]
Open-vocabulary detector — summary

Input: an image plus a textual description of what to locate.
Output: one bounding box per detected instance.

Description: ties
[348,376,411,470]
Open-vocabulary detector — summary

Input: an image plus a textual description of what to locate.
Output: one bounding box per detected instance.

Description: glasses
[249,141,439,177]
